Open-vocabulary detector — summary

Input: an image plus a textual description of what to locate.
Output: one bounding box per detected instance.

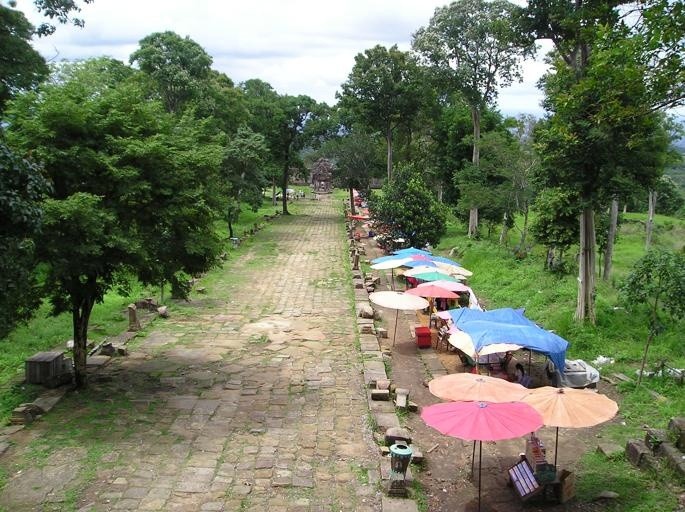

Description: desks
[521,455,560,507]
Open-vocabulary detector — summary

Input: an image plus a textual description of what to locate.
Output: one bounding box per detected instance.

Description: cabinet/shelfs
[24,352,64,385]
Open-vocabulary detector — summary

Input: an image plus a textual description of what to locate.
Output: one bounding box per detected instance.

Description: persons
[513,362,528,383]
[286,188,305,200]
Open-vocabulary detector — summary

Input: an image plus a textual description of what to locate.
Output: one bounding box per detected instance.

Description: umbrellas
[369,246,473,347]
[419,372,619,512]
